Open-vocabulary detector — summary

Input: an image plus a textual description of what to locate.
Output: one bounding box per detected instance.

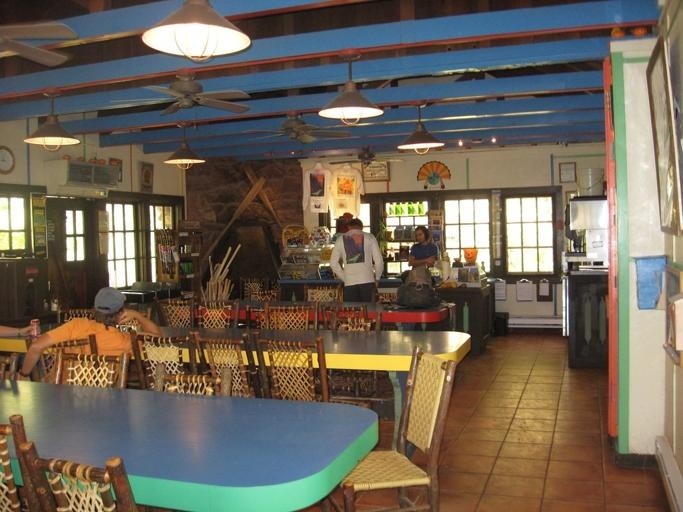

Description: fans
[328,152,403,165]
[145,66,252,115]
[243,118,351,141]
[0,22,79,67]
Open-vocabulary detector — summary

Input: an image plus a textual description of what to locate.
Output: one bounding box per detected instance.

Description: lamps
[397,107,445,155]
[141,0,252,62]
[164,127,206,171]
[317,57,384,127]
[23,91,80,151]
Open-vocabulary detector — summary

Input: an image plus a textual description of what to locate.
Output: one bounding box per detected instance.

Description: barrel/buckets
[573,168,604,196]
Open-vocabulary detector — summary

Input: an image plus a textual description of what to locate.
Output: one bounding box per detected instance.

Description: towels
[636,257,669,308]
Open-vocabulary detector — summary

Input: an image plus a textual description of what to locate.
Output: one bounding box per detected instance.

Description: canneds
[31,319,41,338]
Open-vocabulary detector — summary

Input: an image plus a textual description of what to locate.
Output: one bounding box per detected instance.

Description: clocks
[0,146,15,174]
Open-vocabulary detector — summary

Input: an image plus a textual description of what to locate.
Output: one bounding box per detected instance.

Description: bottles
[481,261,485,271]
[389,202,425,215]
[179,242,184,254]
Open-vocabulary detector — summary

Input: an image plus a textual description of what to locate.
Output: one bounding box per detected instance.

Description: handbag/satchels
[396,265,441,308]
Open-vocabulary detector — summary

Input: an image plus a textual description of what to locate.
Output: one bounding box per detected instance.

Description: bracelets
[19,368,28,377]
[18,328,20,337]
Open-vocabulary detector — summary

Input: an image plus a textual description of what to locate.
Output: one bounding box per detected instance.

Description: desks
[2,379,378,511]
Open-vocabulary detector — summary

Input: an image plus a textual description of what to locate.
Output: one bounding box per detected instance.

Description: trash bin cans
[490,312,509,336]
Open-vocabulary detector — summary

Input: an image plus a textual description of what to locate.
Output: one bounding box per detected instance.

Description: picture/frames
[558,161,578,182]
[645,32,683,235]
[565,274,609,369]
[663,264,683,367]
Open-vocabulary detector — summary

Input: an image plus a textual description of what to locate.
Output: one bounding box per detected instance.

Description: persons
[0,326,40,337]
[329,218,385,303]
[17,286,162,386]
[407,226,440,271]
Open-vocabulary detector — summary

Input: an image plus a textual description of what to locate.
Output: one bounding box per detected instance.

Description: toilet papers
[442,261,449,283]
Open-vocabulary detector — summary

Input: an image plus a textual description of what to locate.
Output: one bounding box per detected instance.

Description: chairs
[323,347,457,510]
[0,414,41,510]
[53,280,476,408]
[19,442,139,512]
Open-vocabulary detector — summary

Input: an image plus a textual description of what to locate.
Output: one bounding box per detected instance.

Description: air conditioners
[44,160,117,198]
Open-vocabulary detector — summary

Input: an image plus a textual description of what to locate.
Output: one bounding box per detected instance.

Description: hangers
[312,156,323,171]
[342,159,353,171]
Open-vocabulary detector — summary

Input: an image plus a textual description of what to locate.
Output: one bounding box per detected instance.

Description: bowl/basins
[453,258,461,262]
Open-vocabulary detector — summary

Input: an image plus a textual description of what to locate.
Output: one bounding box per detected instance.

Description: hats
[95,287,127,314]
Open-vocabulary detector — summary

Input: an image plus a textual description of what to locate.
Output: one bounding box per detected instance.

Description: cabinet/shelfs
[0,257,49,325]
[383,198,430,262]
